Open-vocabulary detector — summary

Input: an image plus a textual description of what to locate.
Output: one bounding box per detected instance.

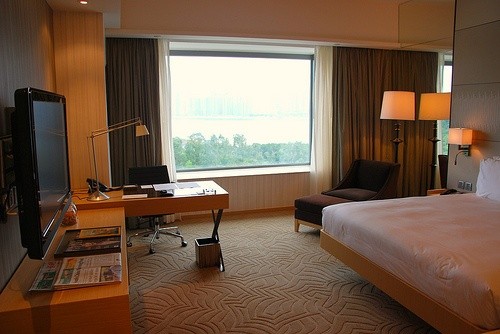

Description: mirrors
[398,0,455,197]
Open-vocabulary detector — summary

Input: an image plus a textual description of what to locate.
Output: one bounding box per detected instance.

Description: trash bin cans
[194,237,220,267]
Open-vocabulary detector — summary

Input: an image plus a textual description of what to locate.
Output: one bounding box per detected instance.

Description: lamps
[380,91,415,197]
[87,117,150,202]
[419,93,451,189]
[448,128,472,165]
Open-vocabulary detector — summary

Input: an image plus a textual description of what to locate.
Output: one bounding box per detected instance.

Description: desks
[0,207,132,334]
[68,180,229,272]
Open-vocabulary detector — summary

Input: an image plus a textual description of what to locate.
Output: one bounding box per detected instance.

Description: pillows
[476,156,500,201]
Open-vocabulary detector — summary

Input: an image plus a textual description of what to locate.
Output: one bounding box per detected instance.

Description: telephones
[86,178,108,193]
[440,188,463,195]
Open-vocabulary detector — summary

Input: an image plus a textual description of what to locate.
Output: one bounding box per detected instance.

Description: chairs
[127,165,188,254]
[294,160,401,232]
[438,155,448,189]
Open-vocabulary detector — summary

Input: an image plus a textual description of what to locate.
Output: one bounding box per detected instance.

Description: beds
[320,192,500,334]
